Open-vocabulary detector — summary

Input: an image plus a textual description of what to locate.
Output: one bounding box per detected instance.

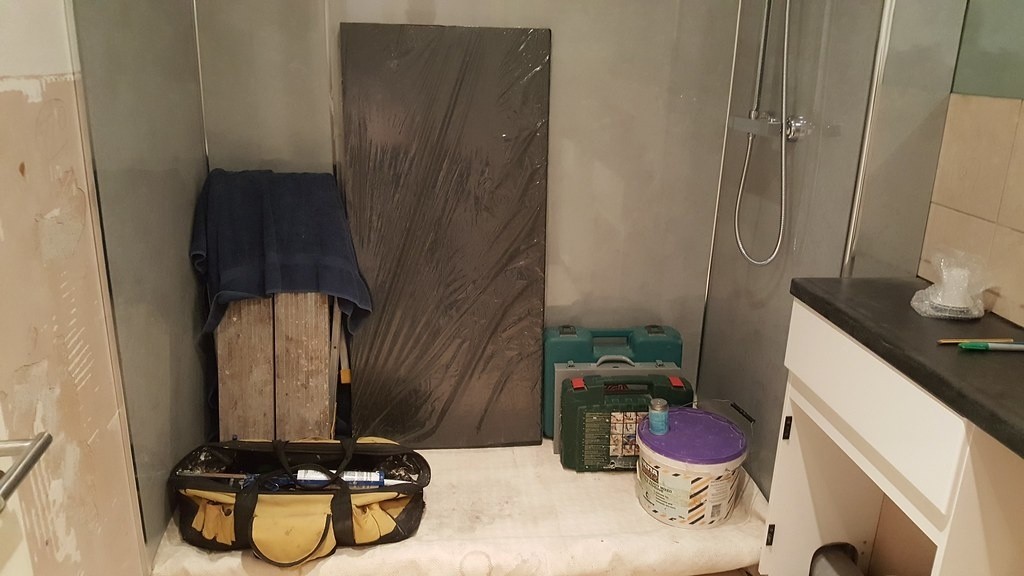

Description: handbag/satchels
[168,435,431,571]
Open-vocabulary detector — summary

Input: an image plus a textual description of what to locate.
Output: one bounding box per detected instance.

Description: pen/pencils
[936,338,1014,345]
[957,343,1024,352]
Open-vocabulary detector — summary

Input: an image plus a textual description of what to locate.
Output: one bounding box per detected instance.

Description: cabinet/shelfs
[757,276,1024,575]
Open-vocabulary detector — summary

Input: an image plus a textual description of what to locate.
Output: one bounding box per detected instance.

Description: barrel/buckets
[633,398,756,531]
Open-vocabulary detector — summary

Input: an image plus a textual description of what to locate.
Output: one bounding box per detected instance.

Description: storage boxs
[545,324,694,475]
